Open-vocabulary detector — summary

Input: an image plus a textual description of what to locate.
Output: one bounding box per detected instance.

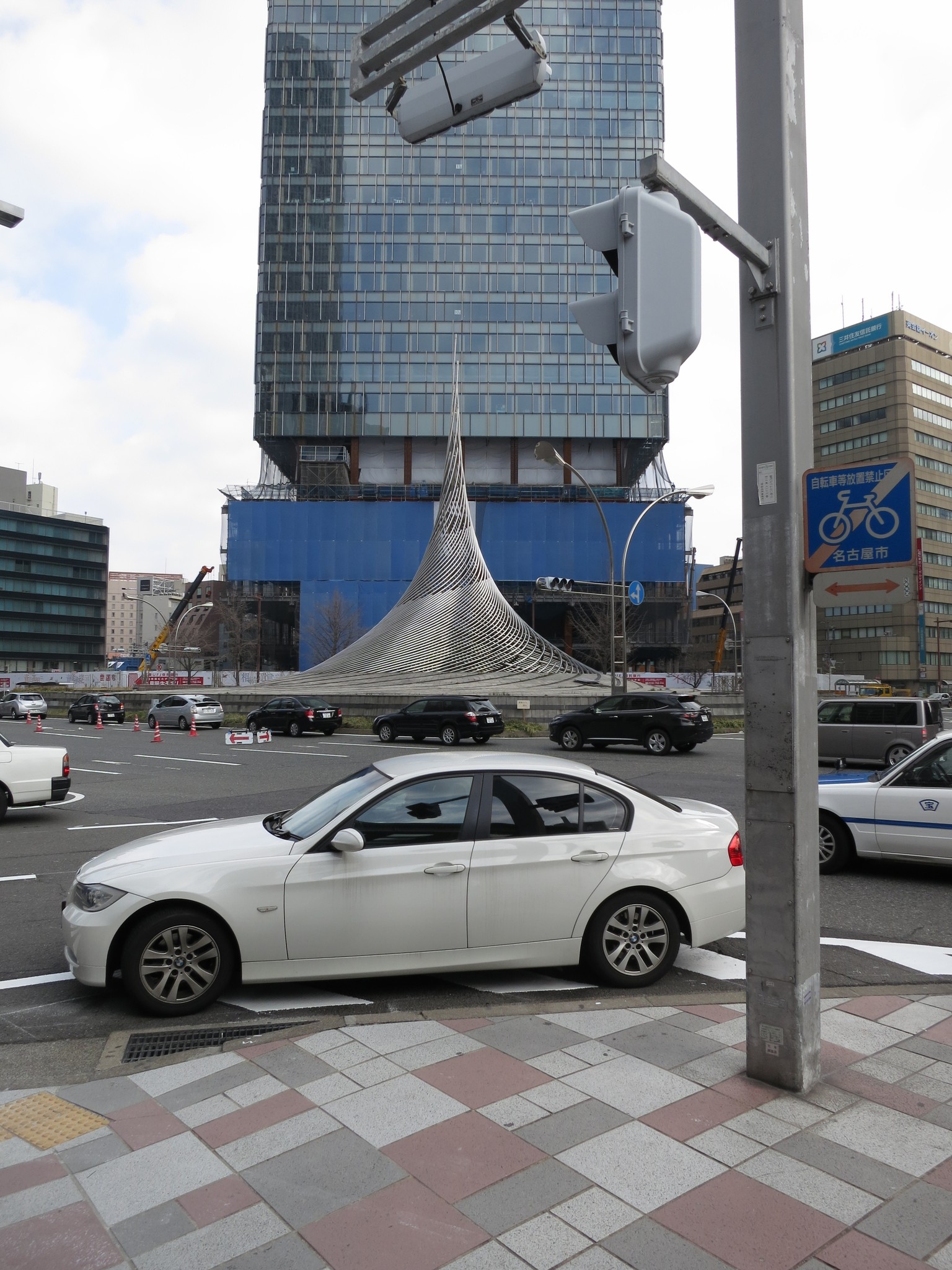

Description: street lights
[692,589,740,694]
[122,591,213,686]
[530,437,711,699]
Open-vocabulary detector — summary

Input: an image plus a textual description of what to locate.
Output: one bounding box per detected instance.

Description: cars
[927,693,951,708]
[0,731,72,819]
[148,694,225,730]
[0,691,48,720]
[57,747,747,1015]
[246,696,343,737]
[818,729,952,875]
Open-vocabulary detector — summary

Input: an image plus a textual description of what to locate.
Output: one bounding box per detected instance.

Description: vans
[818,695,946,771]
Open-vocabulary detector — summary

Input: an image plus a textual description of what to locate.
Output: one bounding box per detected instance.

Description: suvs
[371,695,505,746]
[67,692,125,725]
[547,692,714,756]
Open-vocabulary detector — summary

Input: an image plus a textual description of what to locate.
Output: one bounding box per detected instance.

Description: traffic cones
[186,716,200,736]
[25,709,33,724]
[33,715,44,733]
[150,721,164,742]
[132,714,141,732]
[95,712,105,729]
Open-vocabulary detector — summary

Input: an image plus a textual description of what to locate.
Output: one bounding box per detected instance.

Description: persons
[949,691,952,696]
[912,692,917,697]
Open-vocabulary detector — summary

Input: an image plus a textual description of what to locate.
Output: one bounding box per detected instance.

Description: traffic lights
[562,181,705,396]
[151,648,160,652]
[543,574,575,593]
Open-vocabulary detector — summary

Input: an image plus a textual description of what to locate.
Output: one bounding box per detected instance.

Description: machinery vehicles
[106,564,214,671]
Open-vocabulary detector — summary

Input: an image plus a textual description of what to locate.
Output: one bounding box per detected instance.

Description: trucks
[855,684,913,698]
